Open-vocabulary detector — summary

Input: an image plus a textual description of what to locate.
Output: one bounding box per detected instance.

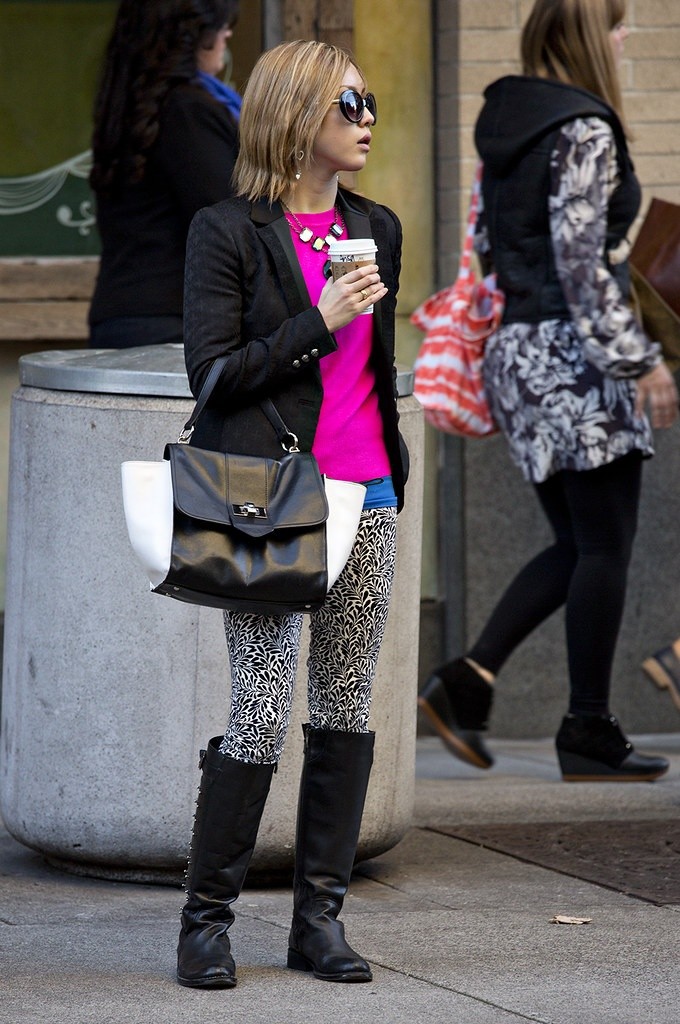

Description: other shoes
[643,640,679,707]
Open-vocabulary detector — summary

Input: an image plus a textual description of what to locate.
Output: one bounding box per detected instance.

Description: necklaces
[280,196,345,280]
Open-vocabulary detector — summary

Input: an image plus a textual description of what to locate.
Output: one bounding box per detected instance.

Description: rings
[361,291,367,299]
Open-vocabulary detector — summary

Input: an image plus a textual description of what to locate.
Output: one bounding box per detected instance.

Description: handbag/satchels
[412,162,500,438]
[120,356,367,614]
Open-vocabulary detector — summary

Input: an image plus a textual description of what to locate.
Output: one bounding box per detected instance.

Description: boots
[416,656,496,768]
[287,723,377,981]
[554,715,670,781]
[177,735,279,989]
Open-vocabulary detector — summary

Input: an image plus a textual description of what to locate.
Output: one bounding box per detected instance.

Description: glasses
[316,89,378,127]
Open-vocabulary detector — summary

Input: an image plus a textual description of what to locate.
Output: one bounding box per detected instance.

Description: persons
[417,1,680,783]
[178,42,410,988]
[91,0,244,351]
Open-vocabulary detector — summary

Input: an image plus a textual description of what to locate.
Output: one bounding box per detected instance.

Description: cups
[328,238,378,314]
